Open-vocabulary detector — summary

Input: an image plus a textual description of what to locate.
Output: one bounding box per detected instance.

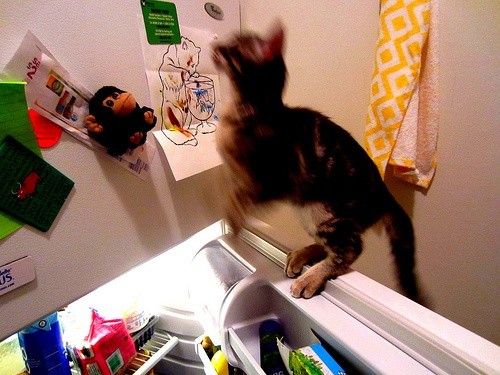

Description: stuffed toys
[84,86,157,157]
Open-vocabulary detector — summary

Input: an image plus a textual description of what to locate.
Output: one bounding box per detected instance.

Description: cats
[206,16,436,314]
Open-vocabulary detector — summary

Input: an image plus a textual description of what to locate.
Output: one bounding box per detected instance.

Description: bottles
[55,90,70,114]
[63,96,76,118]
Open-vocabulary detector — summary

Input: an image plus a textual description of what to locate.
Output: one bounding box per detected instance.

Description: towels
[363,0,440,189]
